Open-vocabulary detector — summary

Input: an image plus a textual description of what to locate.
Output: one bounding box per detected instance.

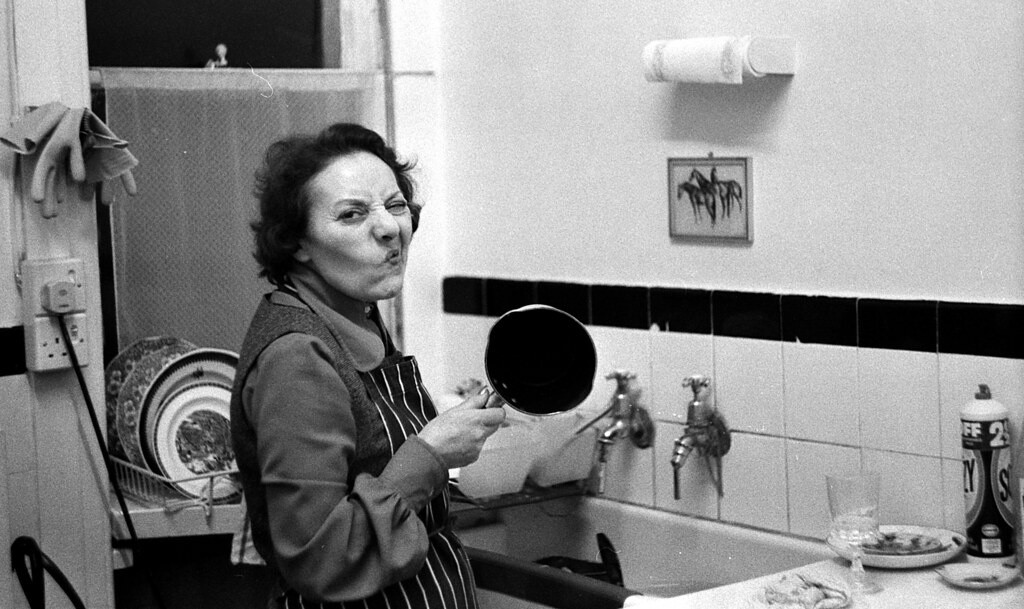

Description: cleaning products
[960,384,1016,570]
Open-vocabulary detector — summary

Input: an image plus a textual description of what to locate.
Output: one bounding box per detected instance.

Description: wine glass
[824,465,885,597]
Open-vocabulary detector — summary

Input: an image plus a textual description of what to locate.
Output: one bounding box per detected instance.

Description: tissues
[640,35,796,86]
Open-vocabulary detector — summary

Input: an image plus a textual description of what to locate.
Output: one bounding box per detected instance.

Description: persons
[230,122,507,609]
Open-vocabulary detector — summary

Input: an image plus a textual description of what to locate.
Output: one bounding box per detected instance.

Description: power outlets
[21,259,88,375]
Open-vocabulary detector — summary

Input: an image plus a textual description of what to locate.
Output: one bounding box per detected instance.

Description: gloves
[1,101,139,219]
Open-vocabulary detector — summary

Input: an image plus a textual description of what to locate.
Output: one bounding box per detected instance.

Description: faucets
[670,375,732,468]
[598,372,654,451]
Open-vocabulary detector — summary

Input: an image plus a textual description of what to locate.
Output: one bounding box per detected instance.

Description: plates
[105,334,243,505]
[933,563,1020,590]
[824,525,968,570]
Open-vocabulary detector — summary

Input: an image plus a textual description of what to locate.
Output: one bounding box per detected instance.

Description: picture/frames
[667,156,755,243]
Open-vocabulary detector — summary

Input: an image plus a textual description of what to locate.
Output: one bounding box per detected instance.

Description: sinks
[451,493,855,599]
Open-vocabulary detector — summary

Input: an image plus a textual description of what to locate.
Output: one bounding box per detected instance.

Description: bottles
[959,380,1021,567]
